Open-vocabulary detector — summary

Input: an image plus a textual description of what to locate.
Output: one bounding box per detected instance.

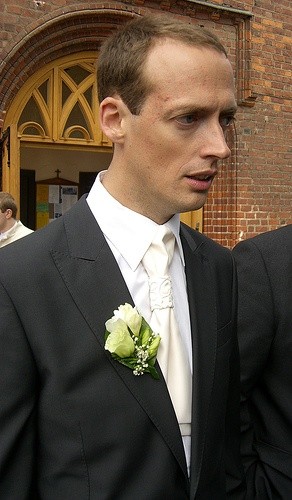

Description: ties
[142,235,193,479]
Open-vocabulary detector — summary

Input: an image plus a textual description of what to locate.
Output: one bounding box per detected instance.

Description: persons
[231,224,292,500]
[0,192,34,249]
[0,14,238,500]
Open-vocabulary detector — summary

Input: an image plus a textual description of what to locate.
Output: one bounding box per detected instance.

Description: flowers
[104,303,162,380]
